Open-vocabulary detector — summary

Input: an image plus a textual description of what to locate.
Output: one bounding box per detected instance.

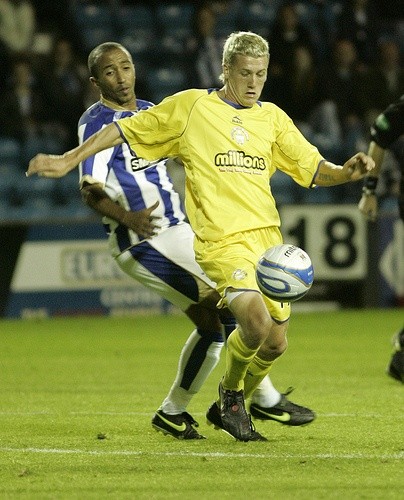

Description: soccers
[254,244,315,303]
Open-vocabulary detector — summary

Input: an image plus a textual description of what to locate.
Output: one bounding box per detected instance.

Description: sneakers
[205,401,270,443]
[249,385,316,427]
[218,375,256,440]
[150,408,207,441]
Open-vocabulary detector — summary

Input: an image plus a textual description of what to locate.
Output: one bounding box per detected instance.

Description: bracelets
[362,187,376,196]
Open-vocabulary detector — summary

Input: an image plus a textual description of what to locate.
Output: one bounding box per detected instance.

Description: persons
[26,32,376,438]
[0,0,403,203]
[359,99,404,384]
[78,42,316,440]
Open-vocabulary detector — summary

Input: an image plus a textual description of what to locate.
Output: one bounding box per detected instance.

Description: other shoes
[387,333,404,384]
